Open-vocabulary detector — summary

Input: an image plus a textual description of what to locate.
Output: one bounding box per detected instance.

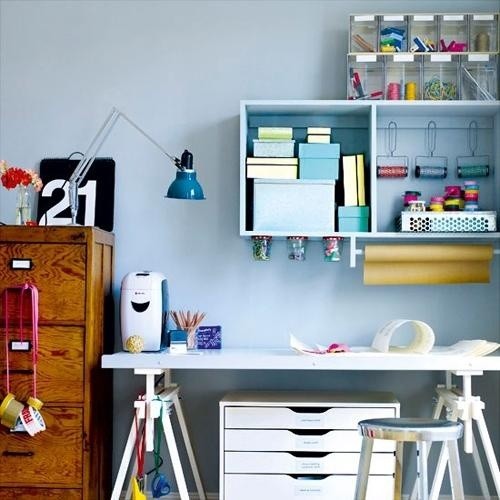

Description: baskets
[394,210,497,233]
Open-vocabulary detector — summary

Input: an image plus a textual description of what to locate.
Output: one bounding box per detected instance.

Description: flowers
[1,159,45,221]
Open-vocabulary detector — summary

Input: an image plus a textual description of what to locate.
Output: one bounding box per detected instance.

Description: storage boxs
[246,122,369,234]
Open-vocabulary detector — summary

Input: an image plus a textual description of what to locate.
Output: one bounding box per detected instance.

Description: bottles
[322,236,344,262]
[285,235,308,262]
[249,236,272,261]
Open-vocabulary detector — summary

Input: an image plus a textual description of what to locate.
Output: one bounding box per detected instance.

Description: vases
[17,186,33,224]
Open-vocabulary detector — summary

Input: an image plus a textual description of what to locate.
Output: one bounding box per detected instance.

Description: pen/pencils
[170,310,206,349]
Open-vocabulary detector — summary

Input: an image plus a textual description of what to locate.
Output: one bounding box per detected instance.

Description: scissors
[151,473,170,500]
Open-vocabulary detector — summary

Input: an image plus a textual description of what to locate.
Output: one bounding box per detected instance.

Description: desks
[98,347,500,499]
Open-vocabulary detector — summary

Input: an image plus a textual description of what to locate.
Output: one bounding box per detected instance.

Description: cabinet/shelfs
[346,12,499,101]
[1,222,116,500]
[237,98,500,243]
[216,388,403,500]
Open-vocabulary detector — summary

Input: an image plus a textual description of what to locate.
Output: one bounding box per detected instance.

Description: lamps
[64,102,206,225]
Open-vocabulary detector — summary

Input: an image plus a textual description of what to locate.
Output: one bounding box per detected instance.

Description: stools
[352,418,468,500]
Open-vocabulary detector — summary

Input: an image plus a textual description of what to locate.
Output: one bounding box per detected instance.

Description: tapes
[0,393,47,437]
